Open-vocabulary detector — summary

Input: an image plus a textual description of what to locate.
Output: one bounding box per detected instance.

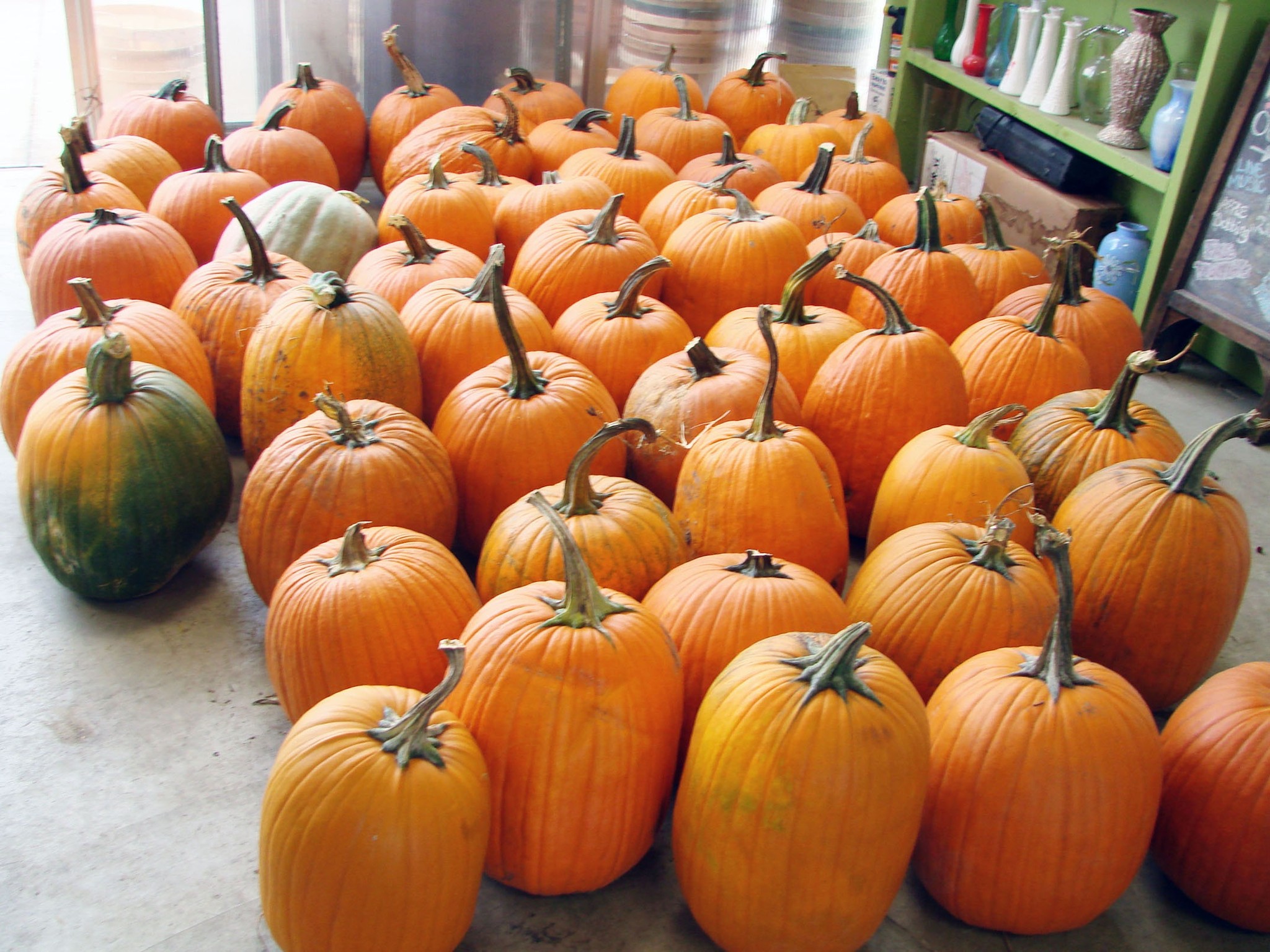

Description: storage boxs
[921,129,1121,289]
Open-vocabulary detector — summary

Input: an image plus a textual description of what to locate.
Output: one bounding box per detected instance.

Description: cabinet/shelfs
[891,1,1270,350]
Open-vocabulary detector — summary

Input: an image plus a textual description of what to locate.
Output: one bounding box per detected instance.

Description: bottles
[936,0,1199,172]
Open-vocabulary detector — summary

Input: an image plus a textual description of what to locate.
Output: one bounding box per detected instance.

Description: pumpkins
[11,41,1267,952]
